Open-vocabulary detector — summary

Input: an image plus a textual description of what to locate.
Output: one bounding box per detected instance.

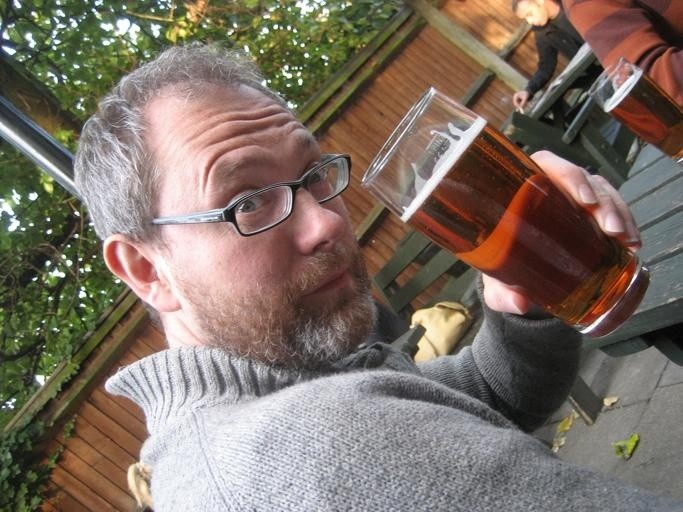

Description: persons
[509,1,615,177]
[561,0,681,121]
[74,46,683,512]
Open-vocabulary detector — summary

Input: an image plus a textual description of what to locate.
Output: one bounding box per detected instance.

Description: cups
[589,58,681,162]
[359,86,651,341]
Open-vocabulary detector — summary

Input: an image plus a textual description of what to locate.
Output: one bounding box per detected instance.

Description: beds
[580,143,683,367]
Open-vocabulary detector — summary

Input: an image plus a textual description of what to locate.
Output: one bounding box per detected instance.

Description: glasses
[151,154,352,237]
